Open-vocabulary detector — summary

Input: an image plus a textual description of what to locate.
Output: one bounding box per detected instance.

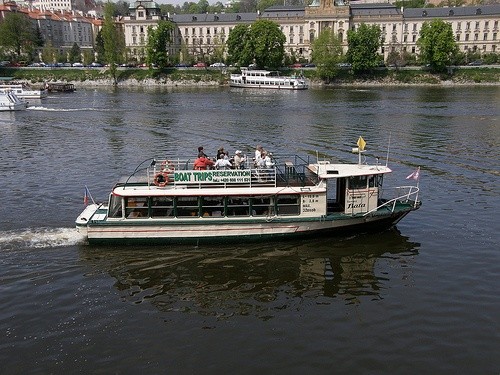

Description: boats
[227,70,308,89]
[0,75,77,112]
[73,133,422,249]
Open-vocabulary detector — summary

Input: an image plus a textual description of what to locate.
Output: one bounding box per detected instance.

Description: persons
[193,147,215,170]
[254,146,275,179]
[214,147,233,170]
[234,150,246,169]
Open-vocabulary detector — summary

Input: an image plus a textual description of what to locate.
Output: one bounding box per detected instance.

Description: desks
[128,211,140,217]
[285,162,293,180]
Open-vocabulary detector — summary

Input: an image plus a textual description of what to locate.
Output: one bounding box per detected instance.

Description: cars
[72,63,84,67]
[388,64,394,67]
[288,62,386,68]
[210,62,227,67]
[0,60,72,67]
[91,62,103,67]
[248,63,258,67]
[467,60,483,66]
[104,62,207,67]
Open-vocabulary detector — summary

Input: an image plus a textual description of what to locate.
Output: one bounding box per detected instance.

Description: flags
[357,136,366,151]
[406,166,420,180]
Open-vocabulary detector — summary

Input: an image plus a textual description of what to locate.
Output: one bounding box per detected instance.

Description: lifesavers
[160,161,173,174]
[153,172,169,186]
